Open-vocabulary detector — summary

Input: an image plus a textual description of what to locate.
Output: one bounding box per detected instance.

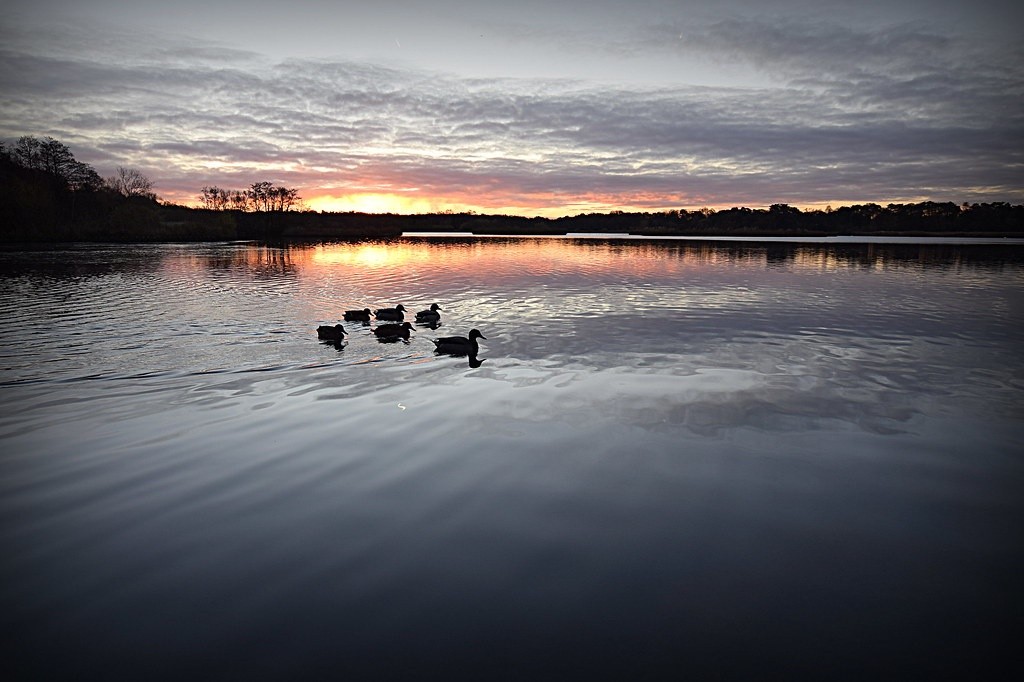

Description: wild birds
[370,321,417,338]
[316,324,349,339]
[373,303,407,322]
[414,303,443,324]
[341,308,374,322]
[432,328,487,353]
